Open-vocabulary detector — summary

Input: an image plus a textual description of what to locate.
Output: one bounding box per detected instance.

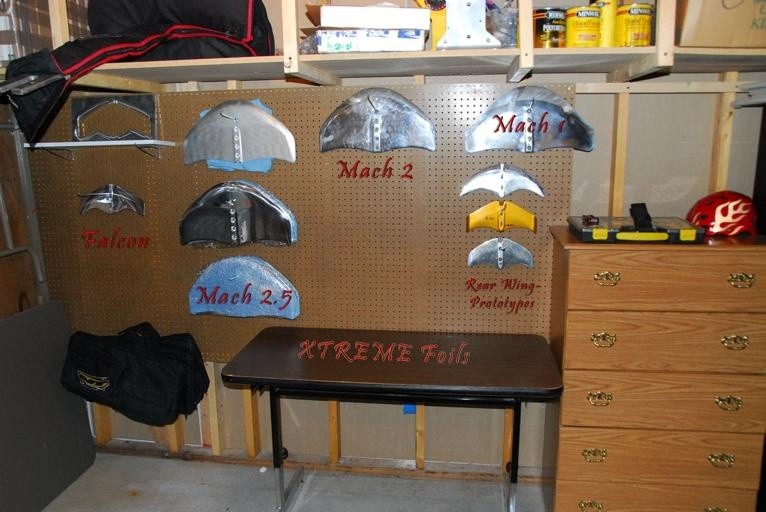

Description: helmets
[686,191,757,246]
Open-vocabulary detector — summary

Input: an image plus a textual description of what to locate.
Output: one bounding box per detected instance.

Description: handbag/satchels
[5,0,275,150]
[60,321,210,427]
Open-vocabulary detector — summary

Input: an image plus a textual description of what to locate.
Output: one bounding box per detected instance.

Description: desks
[220,329,564,512]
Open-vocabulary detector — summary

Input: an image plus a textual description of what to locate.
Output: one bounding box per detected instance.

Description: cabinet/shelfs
[550,218,766,512]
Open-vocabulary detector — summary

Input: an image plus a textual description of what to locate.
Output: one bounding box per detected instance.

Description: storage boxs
[675,0,765,49]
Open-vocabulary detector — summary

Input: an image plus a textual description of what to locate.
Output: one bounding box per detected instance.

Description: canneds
[533,0,655,48]
